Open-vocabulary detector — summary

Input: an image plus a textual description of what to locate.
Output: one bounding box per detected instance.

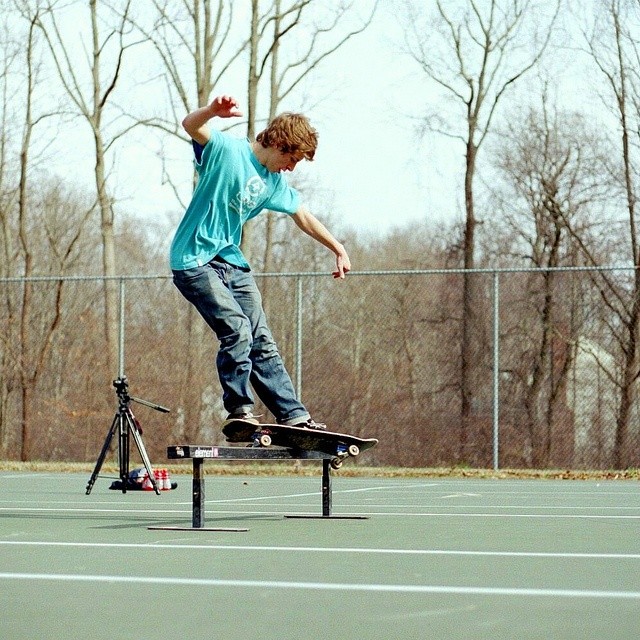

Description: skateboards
[219,418,379,469]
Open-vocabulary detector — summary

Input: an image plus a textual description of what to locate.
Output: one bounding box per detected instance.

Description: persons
[170,95,351,430]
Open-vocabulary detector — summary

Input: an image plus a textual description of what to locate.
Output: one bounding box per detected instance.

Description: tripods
[85,396,170,495]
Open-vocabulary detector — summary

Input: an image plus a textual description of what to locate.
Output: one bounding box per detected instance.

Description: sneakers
[292,419,326,430]
[223,415,264,424]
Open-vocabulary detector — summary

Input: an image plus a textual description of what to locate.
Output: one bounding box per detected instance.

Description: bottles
[163,468,171,490]
[155,470,163,491]
[142,472,153,491]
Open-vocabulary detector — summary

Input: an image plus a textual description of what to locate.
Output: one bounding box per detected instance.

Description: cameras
[114,375,129,395]
